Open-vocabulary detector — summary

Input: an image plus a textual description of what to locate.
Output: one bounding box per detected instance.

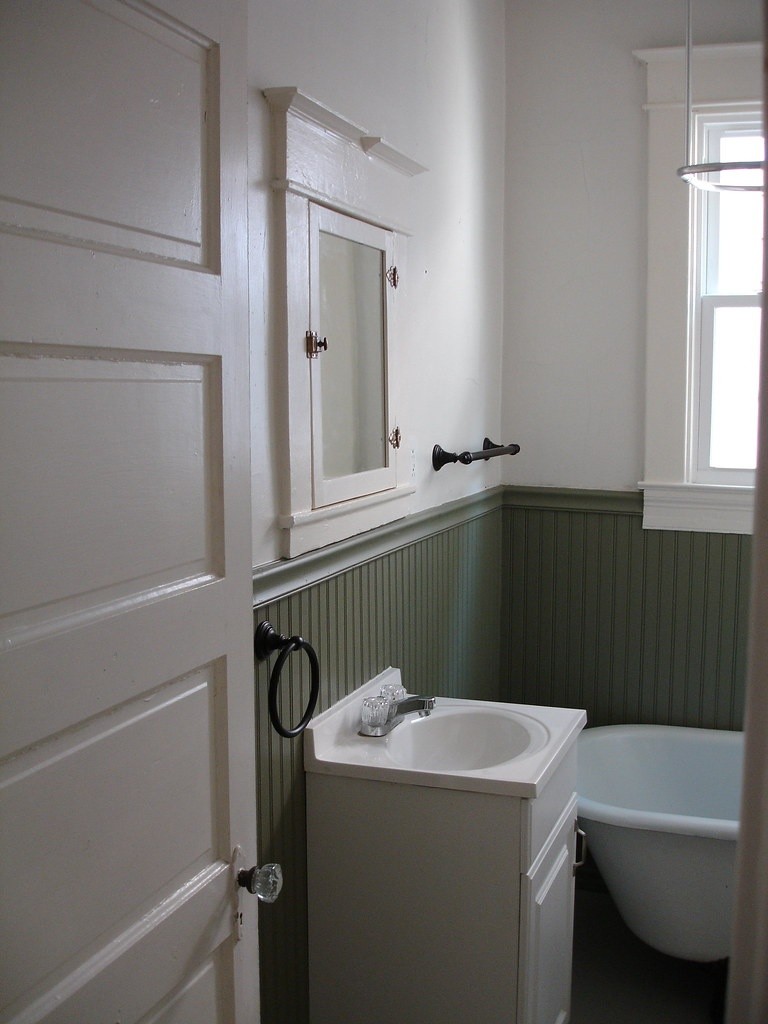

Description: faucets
[388,696,436,720]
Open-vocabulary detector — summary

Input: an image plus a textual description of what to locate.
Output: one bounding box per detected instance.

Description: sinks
[381,700,555,773]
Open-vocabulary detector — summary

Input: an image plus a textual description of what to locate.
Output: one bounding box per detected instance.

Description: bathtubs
[574,722,746,963]
